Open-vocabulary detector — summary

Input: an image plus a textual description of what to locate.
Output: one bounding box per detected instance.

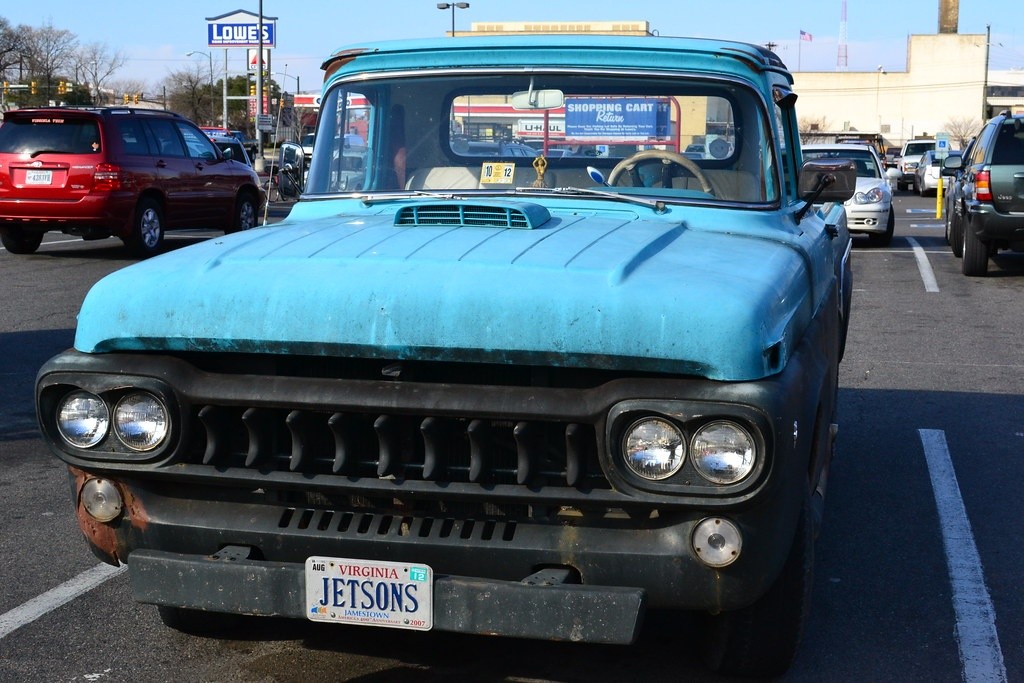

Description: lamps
[877,64,885,73]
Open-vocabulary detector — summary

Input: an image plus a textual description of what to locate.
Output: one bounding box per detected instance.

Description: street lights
[185,49,214,127]
[270,70,300,96]
[437,2,470,38]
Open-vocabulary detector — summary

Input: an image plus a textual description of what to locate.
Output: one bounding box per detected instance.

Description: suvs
[836,133,886,171]
[896,139,952,191]
[941,110,1024,276]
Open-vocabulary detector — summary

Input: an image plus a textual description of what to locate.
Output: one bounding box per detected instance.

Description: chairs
[855,159,867,171]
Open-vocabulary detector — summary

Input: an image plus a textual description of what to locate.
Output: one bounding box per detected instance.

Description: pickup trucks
[35,28,856,675]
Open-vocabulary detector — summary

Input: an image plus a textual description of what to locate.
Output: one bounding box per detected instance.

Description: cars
[914,151,966,198]
[0,104,267,253]
[884,147,904,166]
[799,144,895,246]
[296,129,578,196]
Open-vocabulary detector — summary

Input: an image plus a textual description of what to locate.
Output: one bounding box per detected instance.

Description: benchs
[401,165,760,190]
